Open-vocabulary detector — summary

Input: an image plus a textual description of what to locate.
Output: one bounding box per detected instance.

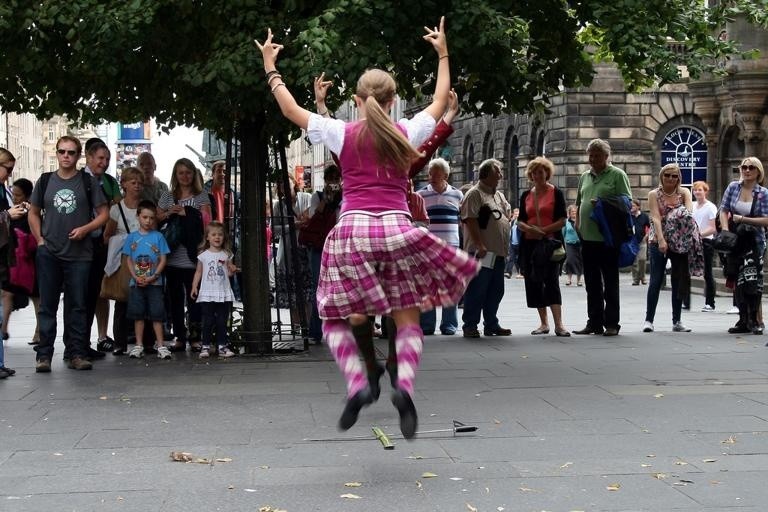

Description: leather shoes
[641,279,647,284]
[632,282,639,285]
[566,281,571,285]
[390,388,418,439]
[88,344,106,358]
[531,325,550,335]
[422,328,435,335]
[484,325,512,337]
[168,342,186,351]
[728,323,752,333]
[337,390,372,434]
[603,325,620,336]
[577,282,582,286]
[26,339,39,346]
[113,343,126,354]
[190,342,202,351]
[442,326,455,337]
[753,324,763,335]
[554,328,571,336]
[572,325,604,335]
[463,326,480,338]
[145,346,157,354]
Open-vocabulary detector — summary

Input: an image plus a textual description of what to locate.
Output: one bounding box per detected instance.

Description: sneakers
[726,306,740,314]
[643,321,655,331]
[156,345,172,360]
[0,370,7,377]
[199,344,211,359]
[218,343,235,358]
[2,366,15,375]
[68,354,92,370]
[34,355,51,371]
[701,304,716,312]
[672,321,691,332]
[129,345,146,359]
[98,337,116,351]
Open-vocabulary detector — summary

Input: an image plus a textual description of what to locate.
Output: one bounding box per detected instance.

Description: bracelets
[435,54,449,61]
[263,71,288,91]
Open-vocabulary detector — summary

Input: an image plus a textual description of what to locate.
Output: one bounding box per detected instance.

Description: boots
[352,323,384,400]
[382,316,397,387]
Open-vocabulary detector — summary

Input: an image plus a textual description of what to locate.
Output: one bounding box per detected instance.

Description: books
[475,250,497,270]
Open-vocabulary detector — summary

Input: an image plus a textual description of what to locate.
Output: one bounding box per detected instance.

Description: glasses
[663,172,679,178]
[741,164,755,171]
[58,149,75,156]
[1,165,13,174]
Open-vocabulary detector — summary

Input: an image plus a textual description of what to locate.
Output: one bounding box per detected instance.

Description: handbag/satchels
[531,186,567,262]
[118,202,131,234]
[714,229,739,254]
[99,253,131,302]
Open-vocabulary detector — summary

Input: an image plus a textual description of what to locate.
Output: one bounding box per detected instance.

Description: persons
[189,220,240,363]
[456,183,477,194]
[80,138,106,175]
[81,146,122,357]
[300,163,344,346]
[137,151,169,231]
[123,200,172,359]
[270,162,315,339]
[516,156,570,338]
[98,168,140,357]
[561,205,586,287]
[0,147,17,379]
[504,206,524,281]
[718,157,768,335]
[630,198,654,285]
[254,15,482,441]
[155,159,211,352]
[28,135,111,374]
[314,71,459,401]
[207,154,237,244]
[677,181,718,314]
[412,156,462,335]
[0,178,40,346]
[574,138,640,337]
[643,163,693,332]
[458,158,513,340]
